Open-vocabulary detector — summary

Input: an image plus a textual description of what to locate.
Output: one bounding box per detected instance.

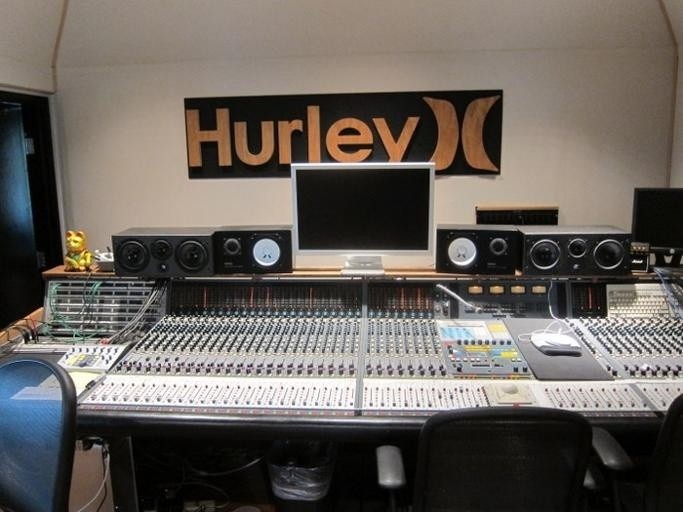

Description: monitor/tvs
[632,187,682,266]
[290,161,437,276]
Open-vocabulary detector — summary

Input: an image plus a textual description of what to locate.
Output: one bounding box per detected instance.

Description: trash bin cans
[265,437,336,512]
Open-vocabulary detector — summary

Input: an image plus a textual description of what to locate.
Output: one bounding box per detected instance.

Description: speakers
[111,226,215,277]
[632,254,649,272]
[212,225,292,274]
[437,223,520,275]
[515,226,634,278]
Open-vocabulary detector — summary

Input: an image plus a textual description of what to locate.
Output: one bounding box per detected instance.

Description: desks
[1,262,682,512]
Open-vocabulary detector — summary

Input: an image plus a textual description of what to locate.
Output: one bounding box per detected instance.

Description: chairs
[370,407,592,510]
[590,395,683,512]
[0,360,75,512]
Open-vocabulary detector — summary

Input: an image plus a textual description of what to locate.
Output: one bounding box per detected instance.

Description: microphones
[431,281,482,314]
